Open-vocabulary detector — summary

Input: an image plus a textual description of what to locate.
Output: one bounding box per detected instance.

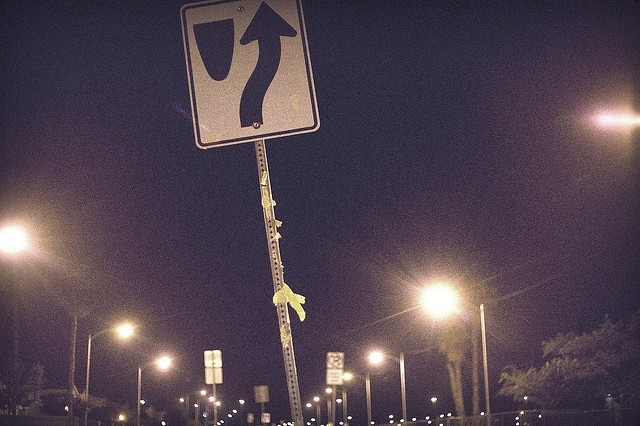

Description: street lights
[418,281,492,426]
[137,354,173,426]
[194,391,221,426]
[311,389,332,425]
[343,373,372,426]
[366,349,407,425]
[84,320,133,426]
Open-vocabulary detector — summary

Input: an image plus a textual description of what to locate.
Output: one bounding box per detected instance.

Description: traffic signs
[179,0,320,150]
